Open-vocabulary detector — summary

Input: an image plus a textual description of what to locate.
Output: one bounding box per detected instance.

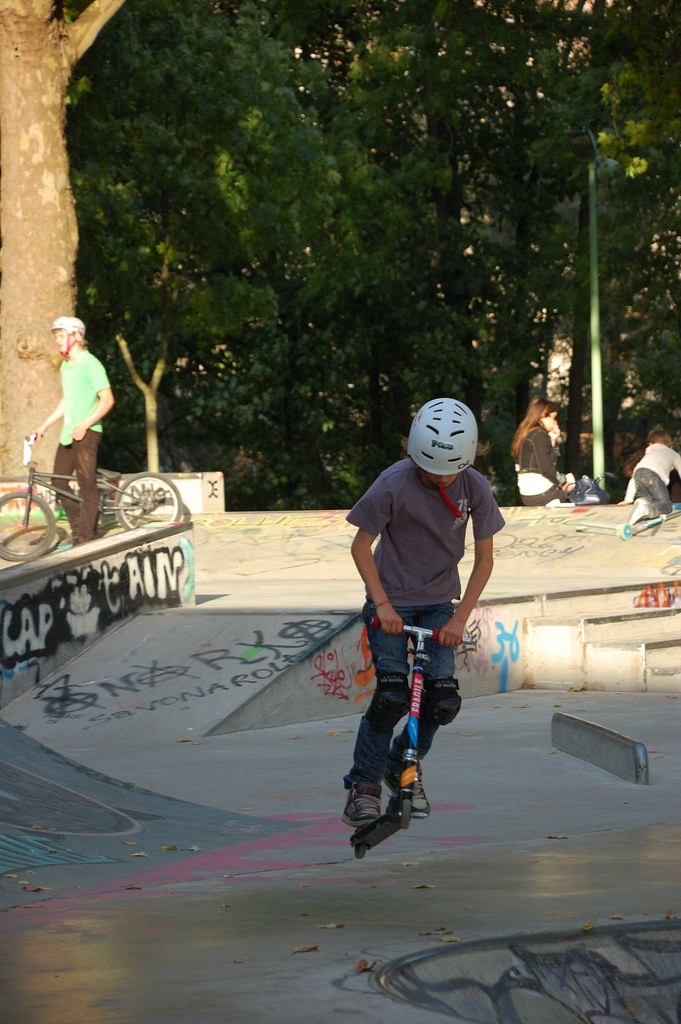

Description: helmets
[51,317,85,336]
[408,398,478,475]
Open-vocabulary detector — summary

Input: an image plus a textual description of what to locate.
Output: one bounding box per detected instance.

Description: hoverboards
[350,615,472,859]
[575,503,681,539]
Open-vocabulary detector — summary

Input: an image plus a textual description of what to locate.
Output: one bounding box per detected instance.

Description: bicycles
[0,433,182,562]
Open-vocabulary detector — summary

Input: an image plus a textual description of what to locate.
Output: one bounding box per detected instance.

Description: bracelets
[374,600,391,609]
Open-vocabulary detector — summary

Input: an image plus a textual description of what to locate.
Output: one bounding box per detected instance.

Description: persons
[512,396,576,507]
[29,316,115,550]
[619,425,681,527]
[343,398,507,826]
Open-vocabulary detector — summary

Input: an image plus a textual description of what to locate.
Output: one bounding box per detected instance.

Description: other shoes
[629,505,649,525]
[635,497,647,504]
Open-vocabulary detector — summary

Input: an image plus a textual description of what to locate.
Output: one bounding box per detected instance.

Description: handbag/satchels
[566,472,609,505]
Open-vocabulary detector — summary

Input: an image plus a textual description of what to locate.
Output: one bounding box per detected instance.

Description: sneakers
[383,750,431,819]
[340,782,381,826]
